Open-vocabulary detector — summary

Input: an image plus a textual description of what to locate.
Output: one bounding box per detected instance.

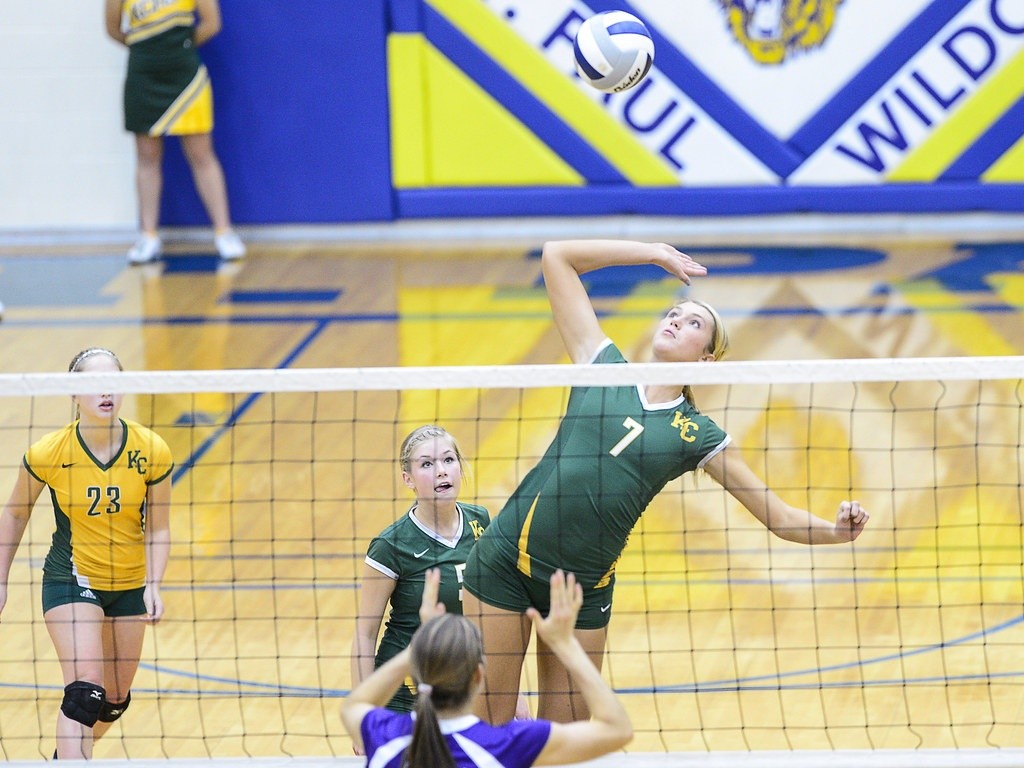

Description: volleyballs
[572,8,656,96]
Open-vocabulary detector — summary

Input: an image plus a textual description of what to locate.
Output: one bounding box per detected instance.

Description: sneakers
[127,236,162,265]
[214,231,247,260]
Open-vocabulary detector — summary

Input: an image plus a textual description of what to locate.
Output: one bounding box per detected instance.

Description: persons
[464,237,870,726]
[105,0,247,263]
[0,349,173,761]
[348,425,490,756]
[342,567,635,767]
